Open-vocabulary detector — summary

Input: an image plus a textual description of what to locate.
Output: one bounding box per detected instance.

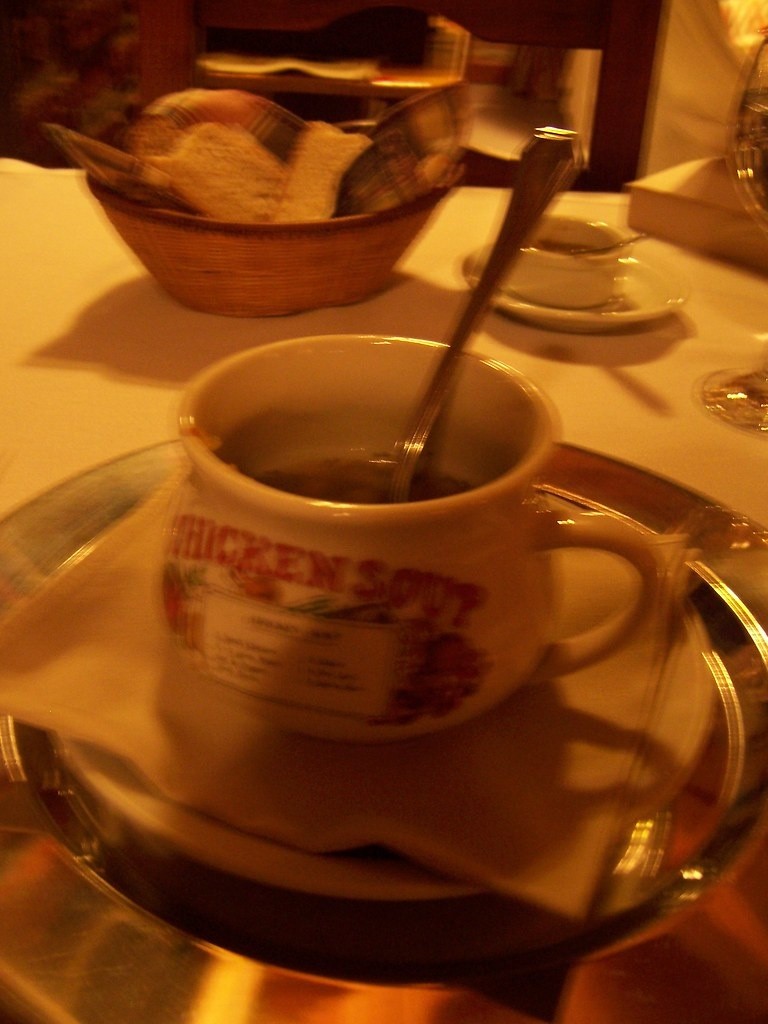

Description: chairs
[133,0,667,188]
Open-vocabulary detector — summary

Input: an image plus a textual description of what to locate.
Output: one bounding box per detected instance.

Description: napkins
[0,468,688,917]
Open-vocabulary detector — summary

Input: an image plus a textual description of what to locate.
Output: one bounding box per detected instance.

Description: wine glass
[692,31,767,440]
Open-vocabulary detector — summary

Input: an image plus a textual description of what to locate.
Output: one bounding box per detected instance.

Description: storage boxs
[624,155,768,274]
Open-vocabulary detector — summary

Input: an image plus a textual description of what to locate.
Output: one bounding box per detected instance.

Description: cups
[155,334,661,745]
[505,216,632,309]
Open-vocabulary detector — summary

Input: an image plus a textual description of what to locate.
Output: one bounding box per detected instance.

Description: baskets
[86,161,466,317]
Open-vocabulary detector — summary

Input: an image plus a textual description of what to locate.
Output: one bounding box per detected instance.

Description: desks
[0,155,768,527]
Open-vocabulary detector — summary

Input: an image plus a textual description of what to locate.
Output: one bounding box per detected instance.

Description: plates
[461,242,689,335]
[0,440,768,1024]
